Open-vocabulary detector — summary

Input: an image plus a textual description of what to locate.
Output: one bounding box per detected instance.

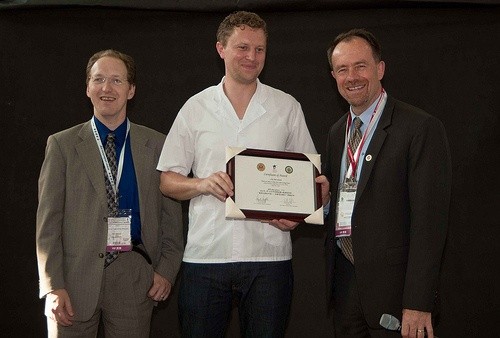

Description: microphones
[380,314,427,337]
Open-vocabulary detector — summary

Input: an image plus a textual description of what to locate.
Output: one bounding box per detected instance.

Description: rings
[417,330,424,333]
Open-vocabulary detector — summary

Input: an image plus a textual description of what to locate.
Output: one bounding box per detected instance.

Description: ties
[338,117,363,266]
[103,133,119,217]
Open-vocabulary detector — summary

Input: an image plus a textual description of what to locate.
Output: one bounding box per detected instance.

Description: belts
[120,236,153,264]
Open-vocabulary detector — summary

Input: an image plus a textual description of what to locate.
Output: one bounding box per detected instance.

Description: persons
[314,28,475,338]
[157,10,320,338]
[35,49,185,338]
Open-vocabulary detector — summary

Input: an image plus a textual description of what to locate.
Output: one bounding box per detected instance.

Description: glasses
[89,75,129,86]
